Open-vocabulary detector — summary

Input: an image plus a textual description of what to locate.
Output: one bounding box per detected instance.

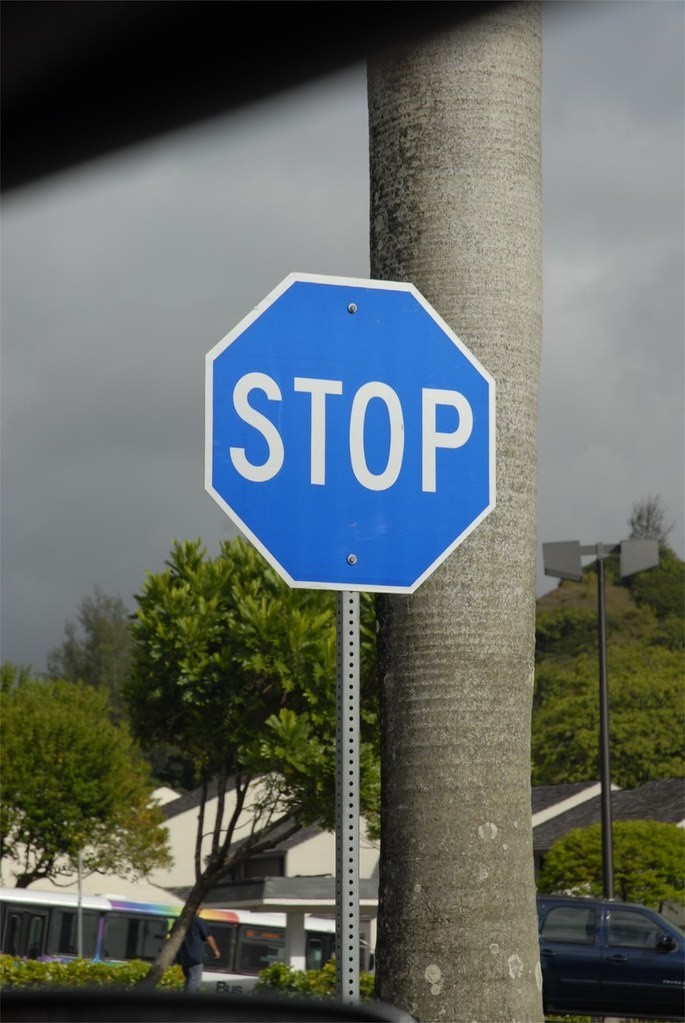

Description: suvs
[535,896,685,1021]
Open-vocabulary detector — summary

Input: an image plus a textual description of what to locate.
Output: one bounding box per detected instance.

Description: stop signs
[200,272,495,595]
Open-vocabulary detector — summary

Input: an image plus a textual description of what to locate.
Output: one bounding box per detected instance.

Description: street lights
[541,537,659,902]
[75,845,95,960]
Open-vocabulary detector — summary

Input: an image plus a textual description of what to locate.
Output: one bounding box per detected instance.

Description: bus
[1,886,375,999]
